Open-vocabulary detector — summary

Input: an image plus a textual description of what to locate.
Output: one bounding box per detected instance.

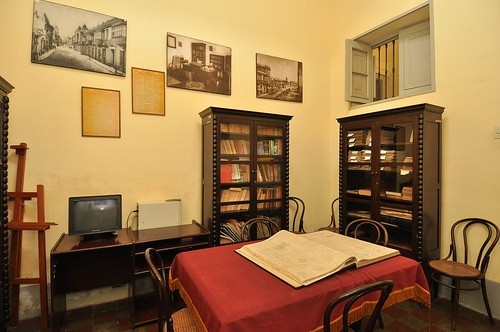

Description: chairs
[289,196,339,234]
[429,218,500,332]
[344,219,388,247]
[324,280,394,332]
[241,219,279,241]
[146,247,199,332]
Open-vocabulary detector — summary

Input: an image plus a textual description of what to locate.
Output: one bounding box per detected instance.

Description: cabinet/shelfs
[199,107,293,246]
[336,103,445,301]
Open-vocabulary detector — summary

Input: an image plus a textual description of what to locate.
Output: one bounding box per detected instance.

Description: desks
[51,220,210,332]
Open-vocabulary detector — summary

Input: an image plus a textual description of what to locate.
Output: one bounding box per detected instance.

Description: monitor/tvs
[68,194,123,241]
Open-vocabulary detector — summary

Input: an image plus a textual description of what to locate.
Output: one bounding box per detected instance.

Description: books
[221,122,285,244]
[235,230,401,288]
[347,129,413,219]
